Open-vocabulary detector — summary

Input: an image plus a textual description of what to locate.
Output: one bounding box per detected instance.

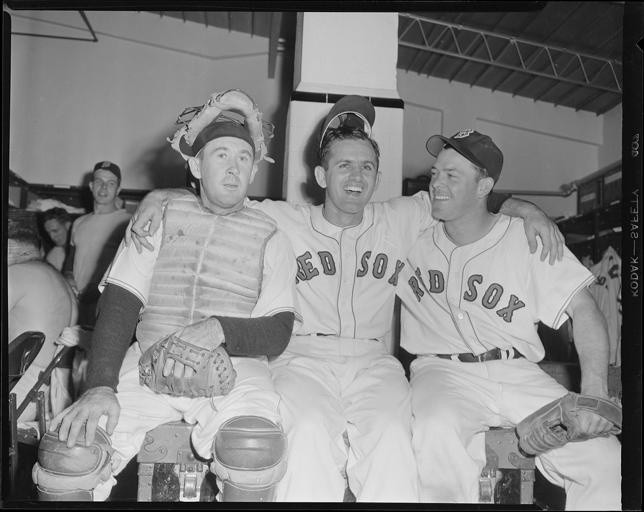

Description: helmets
[170,89,275,162]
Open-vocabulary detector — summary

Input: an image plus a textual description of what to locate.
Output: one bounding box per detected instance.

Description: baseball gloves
[516,393,623,455]
[139,334,237,397]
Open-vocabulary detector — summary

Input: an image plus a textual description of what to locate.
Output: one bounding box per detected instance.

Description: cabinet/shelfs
[557,209,621,276]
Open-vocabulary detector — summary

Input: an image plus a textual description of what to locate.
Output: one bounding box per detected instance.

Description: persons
[31,91,302,503]
[123,96,566,503]
[400,129,621,510]
[8,160,129,425]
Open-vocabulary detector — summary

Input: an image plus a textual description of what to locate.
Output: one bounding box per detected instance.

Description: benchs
[8,172,150,255]
[134,419,539,502]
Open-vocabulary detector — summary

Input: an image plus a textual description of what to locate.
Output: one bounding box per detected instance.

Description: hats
[94,161,121,178]
[425,128,503,181]
[319,96,375,148]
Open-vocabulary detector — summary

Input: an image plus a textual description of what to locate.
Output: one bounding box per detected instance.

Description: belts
[438,346,519,362]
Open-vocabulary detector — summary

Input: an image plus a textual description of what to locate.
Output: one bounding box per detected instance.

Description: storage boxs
[600,166,625,206]
[576,176,602,212]
